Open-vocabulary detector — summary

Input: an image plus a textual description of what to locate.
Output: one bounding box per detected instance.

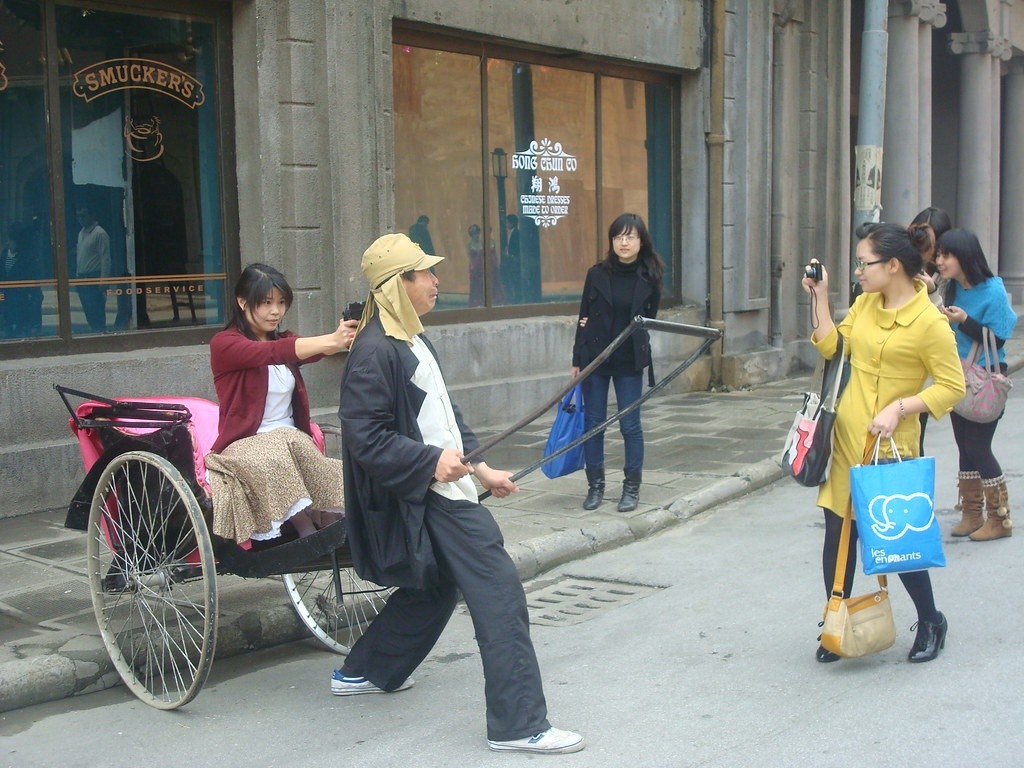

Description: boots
[583,467,606,510]
[969,473,1012,541]
[950,470,985,538]
[616,466,643,512]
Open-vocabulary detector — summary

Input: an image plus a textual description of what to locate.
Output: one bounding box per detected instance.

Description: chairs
[78,394,323,512]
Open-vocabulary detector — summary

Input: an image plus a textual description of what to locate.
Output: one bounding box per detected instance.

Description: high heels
[907,611,947,663]
[815,621,841,662]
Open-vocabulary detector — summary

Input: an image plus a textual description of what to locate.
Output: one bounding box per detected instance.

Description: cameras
[806,262,823,282]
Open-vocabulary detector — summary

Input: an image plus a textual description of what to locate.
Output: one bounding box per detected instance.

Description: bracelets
[899,398,906,420]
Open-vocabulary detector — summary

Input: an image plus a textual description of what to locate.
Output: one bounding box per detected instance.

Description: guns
[342,301,366,349]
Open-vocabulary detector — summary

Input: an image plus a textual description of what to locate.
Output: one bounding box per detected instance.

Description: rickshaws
[47,316,730,714]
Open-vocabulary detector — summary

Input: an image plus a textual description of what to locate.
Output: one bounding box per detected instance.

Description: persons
[409,215,438,303]
[801,224,965,663]
[331,232,584,753]
[467,215,522,306]
[571,213,666,511]
[209,264,359,551]
[0,220,29,336]
[936,227,1020,540]
[76,203,110,332]
[910,206,951,457]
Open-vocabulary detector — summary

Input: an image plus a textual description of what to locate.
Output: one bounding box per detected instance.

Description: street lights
[490,148,512,296]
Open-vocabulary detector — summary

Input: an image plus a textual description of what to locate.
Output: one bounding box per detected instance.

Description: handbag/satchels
[779,331,845,488]
[950,326,1013,423]
[849,426,947,576]
[820,588,896,658]
[540,375,586,479]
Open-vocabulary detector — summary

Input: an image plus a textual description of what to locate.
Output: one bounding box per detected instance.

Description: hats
[348,233,445,353]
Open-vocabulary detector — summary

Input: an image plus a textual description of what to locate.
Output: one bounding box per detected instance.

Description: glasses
[854,255,893,270]
[612,234,643,242]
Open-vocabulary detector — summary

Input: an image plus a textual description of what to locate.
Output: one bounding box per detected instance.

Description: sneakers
[330,666,415,695]
[488,726,584,753]
